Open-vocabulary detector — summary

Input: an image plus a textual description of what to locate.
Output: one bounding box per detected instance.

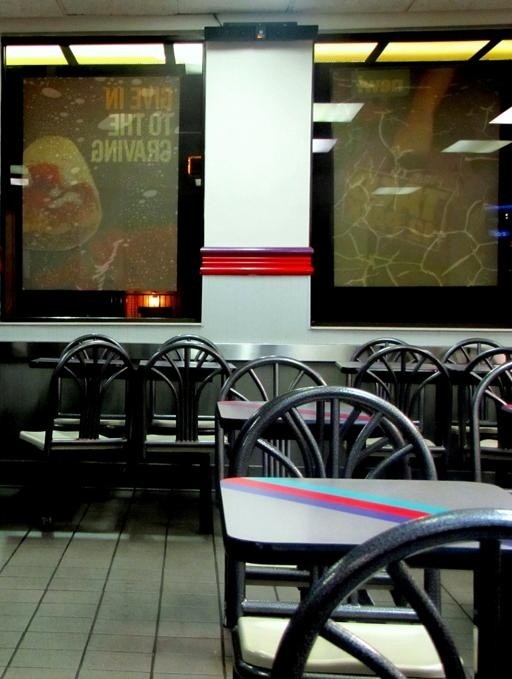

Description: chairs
[134,338,235,536]
[216,355,409,610]
[268,506,510,677]
[441,339,512,442]
[356,345,453,484]
[456,346,510,490]
[469,361,510,499]
[18,337,139,534]
[227,383,468,679]
[346,338,429,427]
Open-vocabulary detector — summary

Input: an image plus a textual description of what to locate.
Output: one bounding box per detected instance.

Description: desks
[216,399,422,442]
[220,476,508,678]
[336,360,511,381]
